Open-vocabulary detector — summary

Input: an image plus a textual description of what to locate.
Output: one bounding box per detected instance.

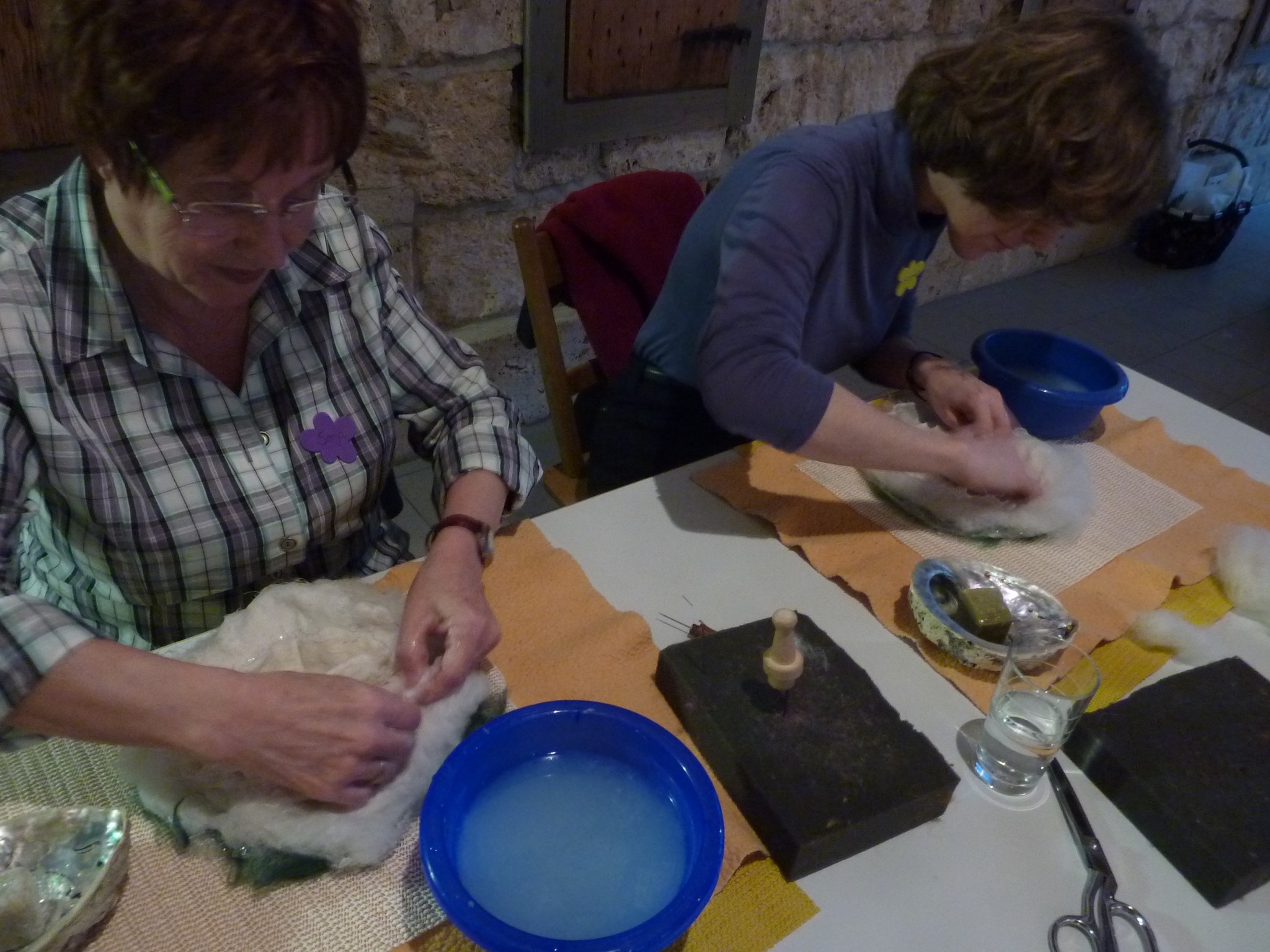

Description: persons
[1,0,545,812]
[582,5,1174,501]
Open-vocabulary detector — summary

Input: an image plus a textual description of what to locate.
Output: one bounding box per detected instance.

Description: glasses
[114,128,358,231]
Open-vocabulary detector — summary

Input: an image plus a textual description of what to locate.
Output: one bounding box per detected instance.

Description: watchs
[423,513,497,568]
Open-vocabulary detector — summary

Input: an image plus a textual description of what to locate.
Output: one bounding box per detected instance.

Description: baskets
[1133,136,1253,267]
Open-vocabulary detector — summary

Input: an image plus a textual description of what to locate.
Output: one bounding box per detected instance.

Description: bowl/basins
[419,700,724,952]
[970,327,1130,442]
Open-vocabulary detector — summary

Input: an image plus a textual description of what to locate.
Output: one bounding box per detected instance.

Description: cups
[964,631,1102,795]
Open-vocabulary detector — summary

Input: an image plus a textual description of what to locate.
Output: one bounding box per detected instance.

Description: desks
[0,343,1269,952]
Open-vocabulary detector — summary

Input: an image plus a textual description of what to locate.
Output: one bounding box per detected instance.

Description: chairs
[514,173,733,508]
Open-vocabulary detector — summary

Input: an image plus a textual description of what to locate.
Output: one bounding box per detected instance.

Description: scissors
[1046,756,1159,952]
